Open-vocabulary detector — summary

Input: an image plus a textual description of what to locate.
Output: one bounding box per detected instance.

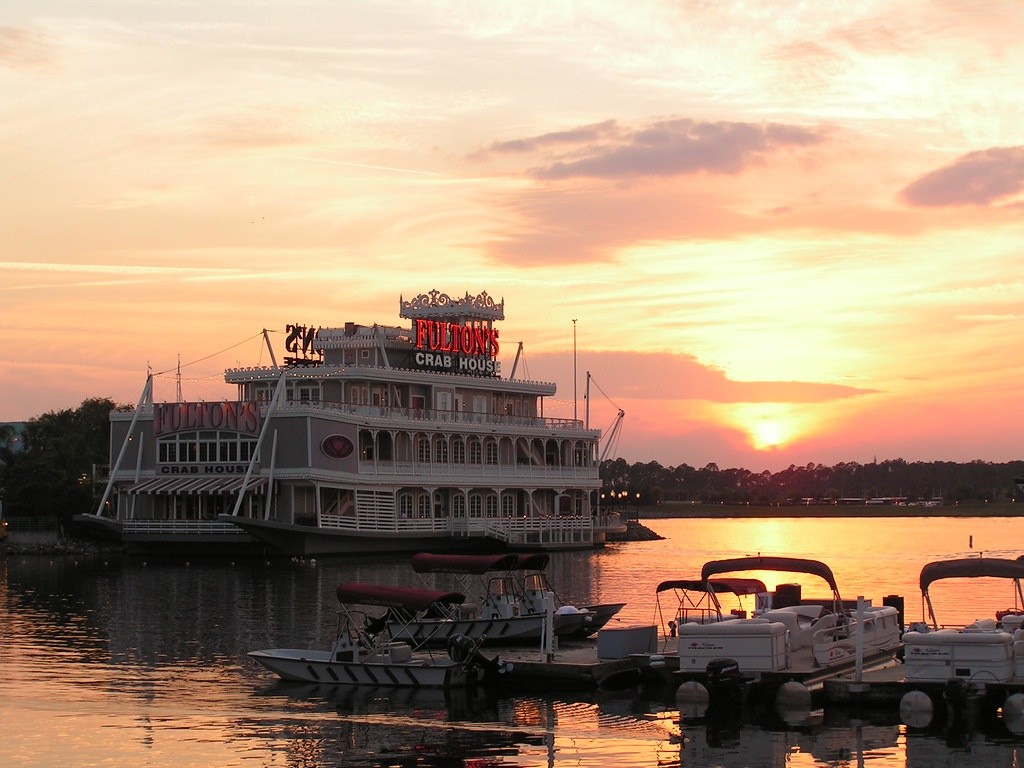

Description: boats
[678,555,904,692]
[72,287,630,561]
[245,579,513,690]
[630,577,769,688]
[900,558,1024,719]
[366,551,625,646]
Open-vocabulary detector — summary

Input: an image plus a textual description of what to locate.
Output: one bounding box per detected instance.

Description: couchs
[679,618,790,672]
[757,605,838,652]
[850,606,899,653]
[964,619,996,632]
[901,627,1014,683]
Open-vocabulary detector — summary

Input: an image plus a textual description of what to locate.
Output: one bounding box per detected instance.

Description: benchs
[381,642,423,664]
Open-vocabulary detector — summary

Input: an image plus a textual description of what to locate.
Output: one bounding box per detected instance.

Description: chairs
[1012,628,1024,679]
